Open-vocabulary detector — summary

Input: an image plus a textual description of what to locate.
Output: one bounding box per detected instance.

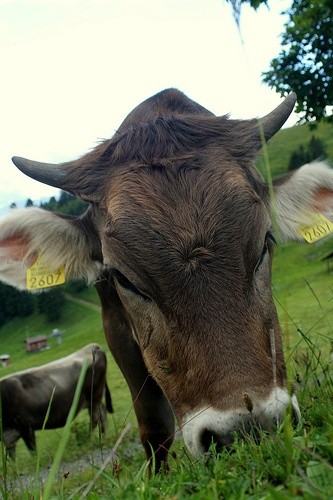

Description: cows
[1,87,333,478]
[1,342,114,466]
